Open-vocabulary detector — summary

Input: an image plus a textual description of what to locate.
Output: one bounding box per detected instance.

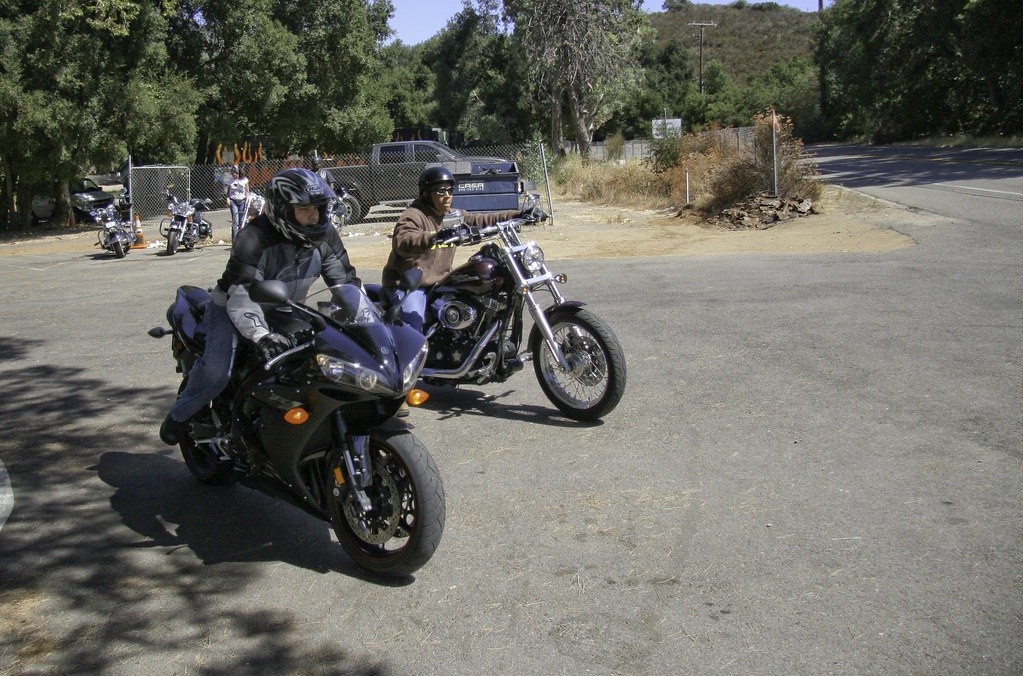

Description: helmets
[119,198,132,212]
[266,167,339,248]
[312,157,323,167]
[419,167,455,198]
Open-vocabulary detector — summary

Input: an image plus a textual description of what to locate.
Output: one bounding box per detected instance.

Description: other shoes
[160,413,185,446]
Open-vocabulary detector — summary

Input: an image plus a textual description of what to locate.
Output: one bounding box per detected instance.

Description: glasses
[428,187,455,196]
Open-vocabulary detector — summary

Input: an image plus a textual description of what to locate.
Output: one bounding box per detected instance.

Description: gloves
[256,331,291,371]
[437,225,460,247]
[521,206,547,225]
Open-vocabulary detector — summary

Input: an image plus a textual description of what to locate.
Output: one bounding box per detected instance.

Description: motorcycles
[148,268,446,580]
[325,186,357,236]
[317,182,628,422]
[159,190,213,255]
[88,197,138,259]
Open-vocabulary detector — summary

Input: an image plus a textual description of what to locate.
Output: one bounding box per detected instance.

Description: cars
[31,177,114,227]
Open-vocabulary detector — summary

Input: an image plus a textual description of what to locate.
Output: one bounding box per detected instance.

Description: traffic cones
[128,215,148,249]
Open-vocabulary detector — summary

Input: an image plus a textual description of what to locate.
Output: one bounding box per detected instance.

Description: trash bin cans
[426,161,520,212]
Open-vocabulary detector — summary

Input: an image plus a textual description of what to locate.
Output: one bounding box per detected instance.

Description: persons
[160,168,368,447]
[225,165,250,246]
[309,157,337,191]
[383,166,547,333]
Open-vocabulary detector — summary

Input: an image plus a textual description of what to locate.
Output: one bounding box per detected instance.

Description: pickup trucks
[322,138,525,226]
